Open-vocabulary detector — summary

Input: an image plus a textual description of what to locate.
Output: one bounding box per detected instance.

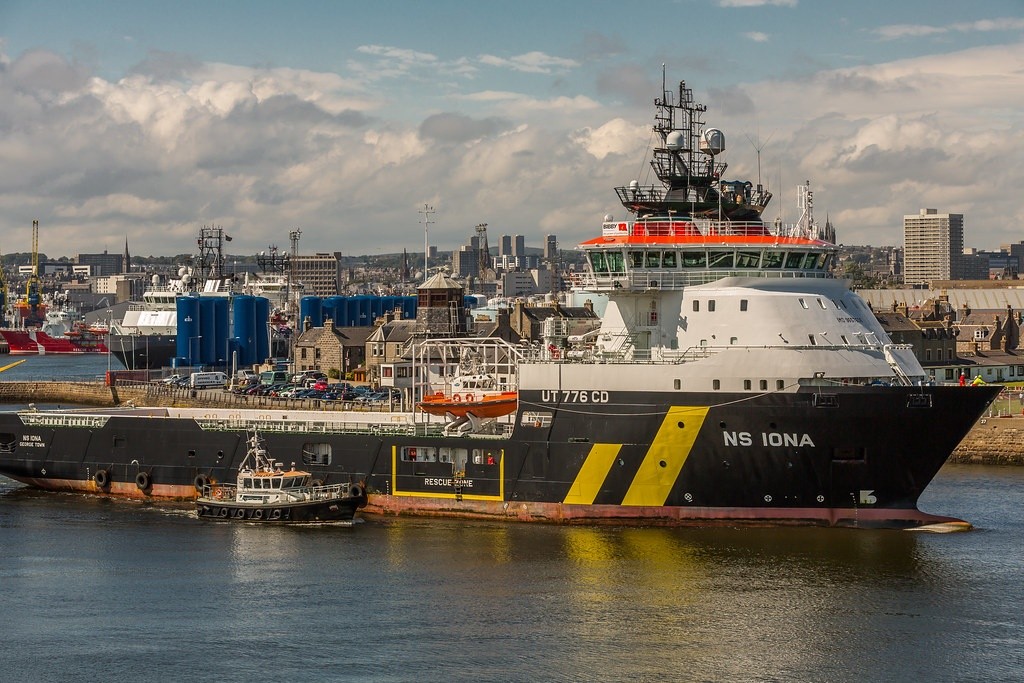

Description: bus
[260,372,289,386]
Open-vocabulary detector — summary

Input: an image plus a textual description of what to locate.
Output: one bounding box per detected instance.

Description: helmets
[978,375,982,378]
[488,453,491,456]
[961,372,965,375]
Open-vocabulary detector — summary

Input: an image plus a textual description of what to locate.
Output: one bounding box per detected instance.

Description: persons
[960,372,966,386]
[548,343,558,357]
[967,375,986,386]
[488,453,495,464]
[411,449,416,455]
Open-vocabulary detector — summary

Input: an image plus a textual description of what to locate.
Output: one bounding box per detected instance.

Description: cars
[232,370,401,405]
[163,374,190,390]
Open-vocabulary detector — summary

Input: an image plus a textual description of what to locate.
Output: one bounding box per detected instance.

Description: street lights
[226,337,241,388]
[189,336,202,376]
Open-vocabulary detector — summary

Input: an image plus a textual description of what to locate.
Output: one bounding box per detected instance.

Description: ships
[2,59,1007,531]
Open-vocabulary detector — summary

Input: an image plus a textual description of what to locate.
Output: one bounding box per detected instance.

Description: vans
[191,372,230,389]
[236,369,258,384]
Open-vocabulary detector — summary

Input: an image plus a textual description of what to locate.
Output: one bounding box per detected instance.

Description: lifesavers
[273,509,282,519]
[466,394,473,404]
[310,479,325,495]
[453,394,461,402]
[95,470,109,488]
[136,472,150,490]
[255,509,264,519]
[194,473,210,492]
[349,484,363,498]
[238,508,246,519]
[220,507,229,517]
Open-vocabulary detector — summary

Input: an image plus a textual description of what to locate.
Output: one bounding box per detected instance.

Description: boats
[0,219,136,356]
[416,367,519,422]
[103,266,304,369]
[196,421,368,524]
